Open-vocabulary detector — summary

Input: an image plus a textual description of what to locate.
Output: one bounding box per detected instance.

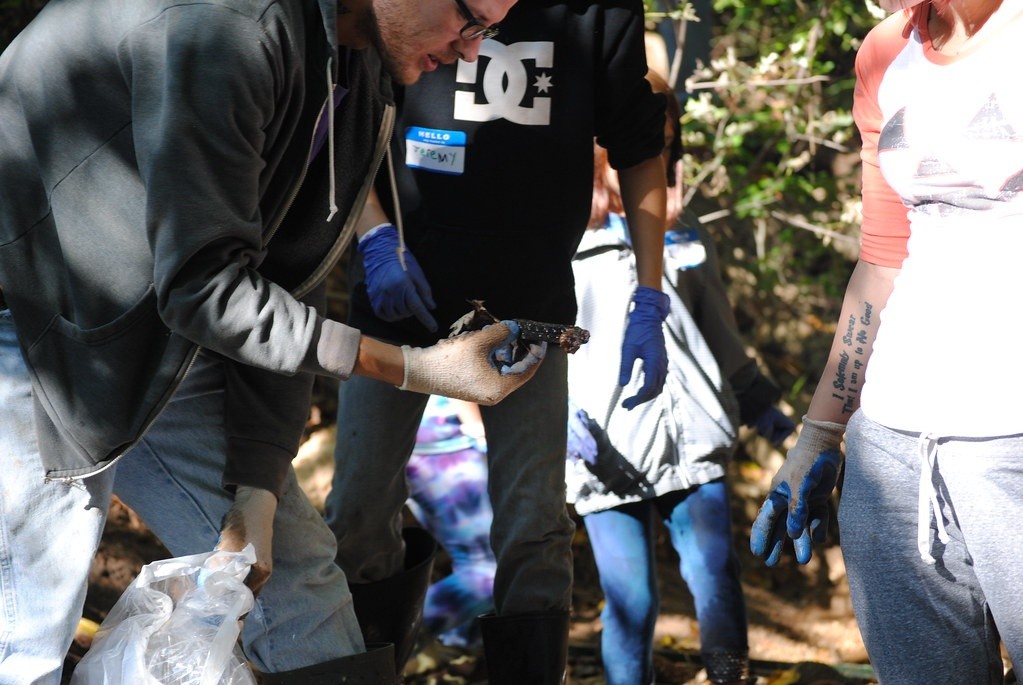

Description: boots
[478,609,565,685]
[349,526,439,685]
[259,645,395,685]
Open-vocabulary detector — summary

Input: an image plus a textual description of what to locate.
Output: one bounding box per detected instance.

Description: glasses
[456,0,499,42]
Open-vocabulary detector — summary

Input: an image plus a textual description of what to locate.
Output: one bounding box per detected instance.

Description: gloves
[359,222,440,333]
[749,416,845,568]
[394,321,549,407]
[747,406,794,446]
[614,287,672,409]
[200,484,279,594]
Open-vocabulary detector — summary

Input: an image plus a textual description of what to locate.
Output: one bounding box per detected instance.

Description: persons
[401,393,500,685]
[748,0,1023,685]
[0,0,550,685]
[560,65,780,685]
[318,0,670,685]
